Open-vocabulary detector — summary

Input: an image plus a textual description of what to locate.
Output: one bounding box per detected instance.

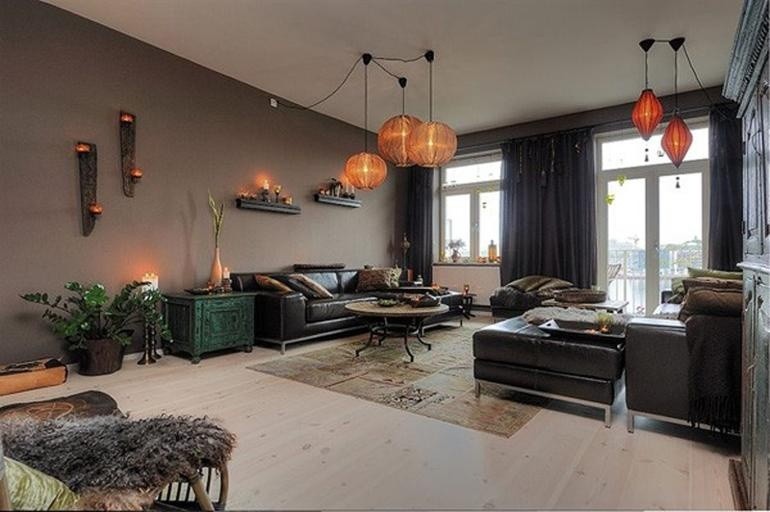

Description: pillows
[286,273,334,299]
[669,265,743,321]
[506,274,551,292]
[354,267,393,293]
[362,264,403,290]
[268,274,322,300]
[254,273,308,301]
[538,278,574,294]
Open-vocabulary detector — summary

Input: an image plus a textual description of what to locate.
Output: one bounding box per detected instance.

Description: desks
[538,296,630,314]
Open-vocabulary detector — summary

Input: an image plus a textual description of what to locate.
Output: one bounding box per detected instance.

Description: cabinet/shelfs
[737,272,770,509]
[159,289,259,365]
[722,1,769,255]
[237,192,364,216]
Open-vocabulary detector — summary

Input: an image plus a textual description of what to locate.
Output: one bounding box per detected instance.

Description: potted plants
[16,279,177,374]
[207,192,227,287]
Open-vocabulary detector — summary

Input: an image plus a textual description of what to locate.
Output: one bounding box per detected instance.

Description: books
[0,357,68,395]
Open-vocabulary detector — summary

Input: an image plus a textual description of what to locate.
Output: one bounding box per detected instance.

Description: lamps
[76,141,103,238]
[631,34,694,188]
[344,49,457,190]
[119,112,144,198]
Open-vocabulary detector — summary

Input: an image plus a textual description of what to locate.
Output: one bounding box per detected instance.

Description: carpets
[244,325,554,438]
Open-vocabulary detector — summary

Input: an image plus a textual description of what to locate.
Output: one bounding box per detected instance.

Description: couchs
[489,285,553,318]
[473,313,623,427]
[624,287,748,437]
[231,263,468,355]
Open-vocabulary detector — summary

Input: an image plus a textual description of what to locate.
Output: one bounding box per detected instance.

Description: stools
[462,294,477,318]
